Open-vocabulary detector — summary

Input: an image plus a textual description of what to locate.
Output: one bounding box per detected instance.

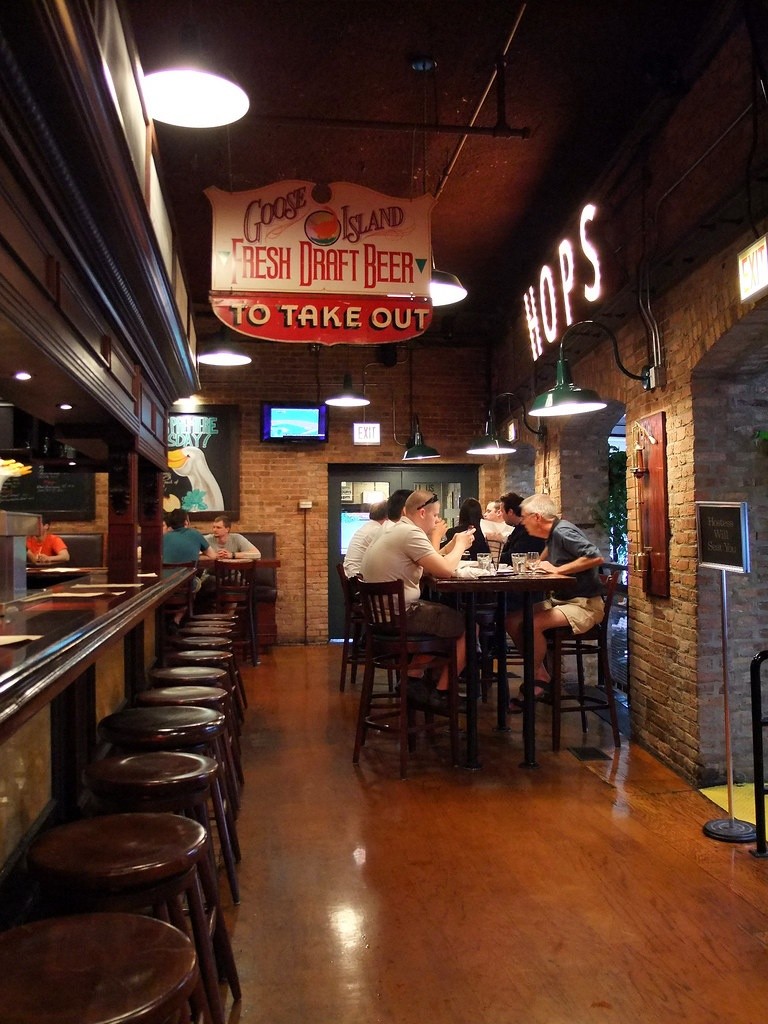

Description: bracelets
[232,552,235,558]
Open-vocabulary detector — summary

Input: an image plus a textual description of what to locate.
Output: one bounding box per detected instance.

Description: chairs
[164,557,258,667]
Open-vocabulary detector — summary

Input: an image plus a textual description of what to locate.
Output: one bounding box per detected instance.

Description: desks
[193,559,280,570]
[424,571,577,772]
[26,567,108,577]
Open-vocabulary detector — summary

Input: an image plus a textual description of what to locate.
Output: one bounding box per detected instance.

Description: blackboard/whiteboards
[0,447,93,521]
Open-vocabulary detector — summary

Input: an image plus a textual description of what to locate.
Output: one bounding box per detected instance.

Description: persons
[480,492,605,714]
[27,517,69,562]
[341,490,476,713]
[137,516,173,559]
[446,498,490,655]
[161,510,215,634]
[204,515,261,615]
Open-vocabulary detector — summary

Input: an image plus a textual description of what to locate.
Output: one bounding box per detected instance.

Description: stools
[0,614,248,1024]
[336,552,621,780]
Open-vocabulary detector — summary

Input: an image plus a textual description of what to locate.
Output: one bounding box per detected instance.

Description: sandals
[506,674,556,714]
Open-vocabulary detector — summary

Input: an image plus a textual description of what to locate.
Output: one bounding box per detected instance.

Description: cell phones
[467,526,474,533]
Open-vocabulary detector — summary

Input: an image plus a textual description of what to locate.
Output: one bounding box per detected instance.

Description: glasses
[519,513,535,523]
[416,493,439,511]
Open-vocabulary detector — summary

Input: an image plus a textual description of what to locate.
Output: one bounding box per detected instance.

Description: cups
[526,552,540,574]
[477,553,492,570]
[511,553,527,574]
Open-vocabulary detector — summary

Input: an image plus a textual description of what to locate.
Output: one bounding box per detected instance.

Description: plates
[497,569,513,573]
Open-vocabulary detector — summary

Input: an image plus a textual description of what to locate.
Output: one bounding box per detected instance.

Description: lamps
[140,0,250,131]
[196,324,252,367]
[525,319,651,417]
[325,346,371,408]
[401,412,441,461]
[465,392,546,455]
[410,58,467,308]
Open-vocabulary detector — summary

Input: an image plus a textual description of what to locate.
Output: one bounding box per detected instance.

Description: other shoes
[427,686,468,713]
[394,674,432,707]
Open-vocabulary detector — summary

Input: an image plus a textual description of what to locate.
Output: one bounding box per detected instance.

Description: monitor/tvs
[261,401,329,444]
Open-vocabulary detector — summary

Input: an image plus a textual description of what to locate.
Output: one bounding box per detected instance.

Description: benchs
[198,532,277,654]
[56,533,103,567]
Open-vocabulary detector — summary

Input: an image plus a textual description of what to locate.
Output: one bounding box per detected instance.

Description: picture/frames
[166,403,240,522]
